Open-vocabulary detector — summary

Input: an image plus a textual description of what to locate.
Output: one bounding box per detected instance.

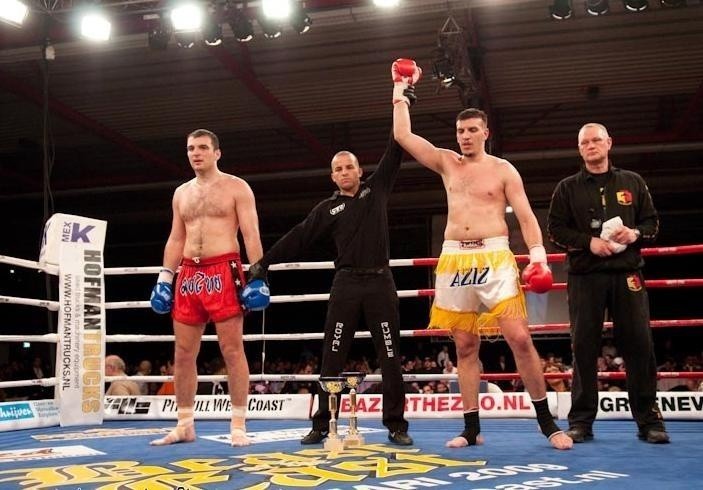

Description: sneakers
[388,429,415,447]
[565,426,596,445]
[301,426,338,444]
[636,426,673,444]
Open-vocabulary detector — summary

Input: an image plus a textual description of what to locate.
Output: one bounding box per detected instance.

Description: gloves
[404,84,419,106]
[521,246,553,296]
[390,58,425,105]
[241,280,271,312]
[150,269,176,316]
[246,263,271,287]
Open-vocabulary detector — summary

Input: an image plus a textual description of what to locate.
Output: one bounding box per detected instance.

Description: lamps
[0,1,702,43]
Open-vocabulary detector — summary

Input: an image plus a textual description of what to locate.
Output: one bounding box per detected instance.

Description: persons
[544,120,672,445]
[247,84,418,445]
[390,58,575,450]
[150,129,273,447]
